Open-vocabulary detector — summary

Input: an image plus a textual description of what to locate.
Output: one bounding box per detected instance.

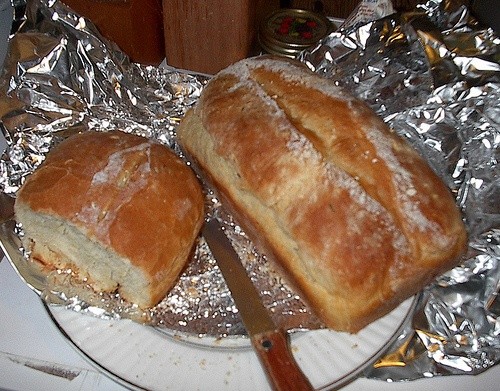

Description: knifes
[201,218,314,391]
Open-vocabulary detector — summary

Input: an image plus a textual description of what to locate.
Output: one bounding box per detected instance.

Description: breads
[12,130,206,311]
[176,54,471,334]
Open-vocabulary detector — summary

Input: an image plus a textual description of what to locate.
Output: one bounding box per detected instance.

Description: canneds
[253,8,336,61]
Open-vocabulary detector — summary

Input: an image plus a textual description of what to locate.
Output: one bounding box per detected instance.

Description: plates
[34,293,419,391]
